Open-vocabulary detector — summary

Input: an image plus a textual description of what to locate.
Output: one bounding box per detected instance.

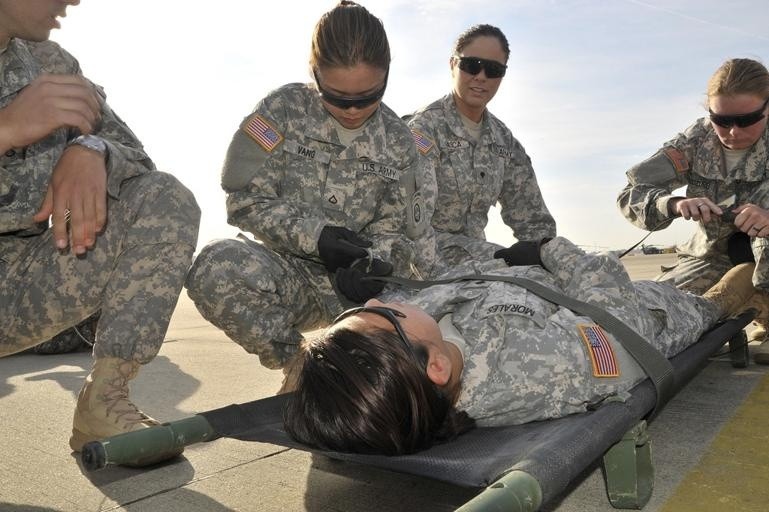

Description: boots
[69,357,184,468]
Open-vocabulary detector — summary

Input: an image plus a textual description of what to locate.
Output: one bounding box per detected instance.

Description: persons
[0,1,208,471]
[282,235,759,455]
[409,23,558,280]
[182,0,421,400]
[617,56,768,366]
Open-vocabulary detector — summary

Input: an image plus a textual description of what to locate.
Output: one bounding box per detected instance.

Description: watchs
[64,133,108,157]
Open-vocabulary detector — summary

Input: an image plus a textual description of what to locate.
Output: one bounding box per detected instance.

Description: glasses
[331,305,426,370]
[455,56,507,78]
[312,67,388,108]
[707,101,769,129]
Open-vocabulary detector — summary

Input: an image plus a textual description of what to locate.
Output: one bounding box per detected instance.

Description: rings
[698,201,705,207]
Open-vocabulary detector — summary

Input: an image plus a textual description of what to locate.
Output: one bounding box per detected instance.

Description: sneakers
[701,262,756,323]
[751,327,769,362]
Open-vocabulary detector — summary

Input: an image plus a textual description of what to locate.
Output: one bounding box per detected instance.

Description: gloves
[494,236,552,272]
[337,259,393,303]
[318,227,374,272]
